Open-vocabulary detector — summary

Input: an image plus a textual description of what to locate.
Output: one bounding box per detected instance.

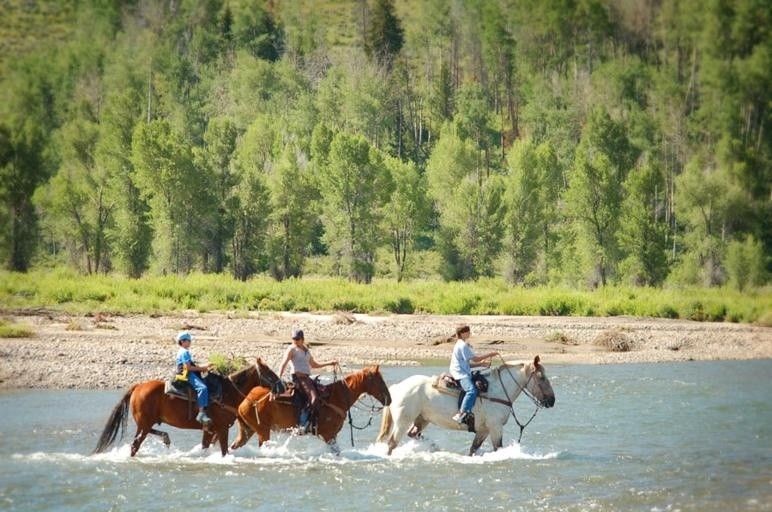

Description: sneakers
[195,412,212,424]
[452,413,471,430]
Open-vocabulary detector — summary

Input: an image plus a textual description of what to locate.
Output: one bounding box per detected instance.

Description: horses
[376,354,556,456]
[230,364,392,457]
[87,356,286,458]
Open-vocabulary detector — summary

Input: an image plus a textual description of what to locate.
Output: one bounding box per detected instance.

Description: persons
[175,333,214,424]
[278,329,338,435]
[451,322,498,421]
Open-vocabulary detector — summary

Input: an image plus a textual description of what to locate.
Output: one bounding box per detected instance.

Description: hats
[178,331,192,342]
[292,328,304,340]
